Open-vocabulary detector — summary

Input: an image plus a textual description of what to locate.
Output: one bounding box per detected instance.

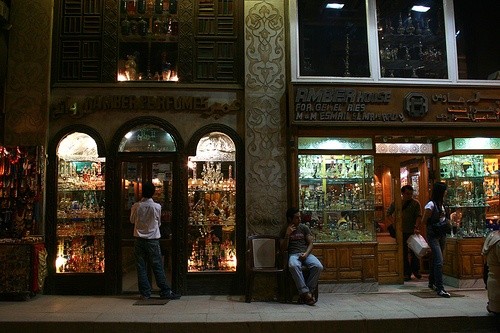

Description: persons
[337,211,348,226]
[421,181,453,298]
[279,207,324,305]
[385,184,423,281]
[130,181,181,300]
[481,220,500,317]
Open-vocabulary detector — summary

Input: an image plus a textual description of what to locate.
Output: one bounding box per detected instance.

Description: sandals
[305,293,317,304]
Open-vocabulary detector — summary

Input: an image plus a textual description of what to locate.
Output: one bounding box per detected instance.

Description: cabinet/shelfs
[188,190,237,273]
[119,13,180,81]
[376,32,446,77]
[299,177,375,242]
[440,154,500,238]
[57,190,105,274]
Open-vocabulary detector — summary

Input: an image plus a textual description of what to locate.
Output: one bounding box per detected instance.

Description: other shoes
[404,275,411,280]
[438,290,450,297]
[161,290,181,299]
[414,271,422,279]
[140,295,149,300]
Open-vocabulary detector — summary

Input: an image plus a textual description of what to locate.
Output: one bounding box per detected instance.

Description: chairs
[246,235,318,303]
